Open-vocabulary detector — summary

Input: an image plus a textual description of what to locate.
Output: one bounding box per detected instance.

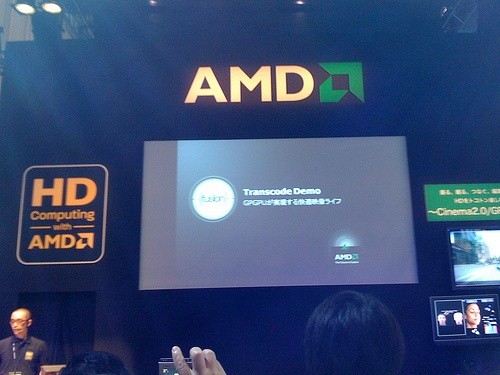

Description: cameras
[158,357,193,375]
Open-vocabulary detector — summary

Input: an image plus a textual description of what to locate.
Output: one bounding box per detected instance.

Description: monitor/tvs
[429,294,500,346]
[447,226,500,291]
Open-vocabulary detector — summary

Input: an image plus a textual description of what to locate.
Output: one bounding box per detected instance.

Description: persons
[464,303,481,328]
[171,346,227,375]
[453,312,463,326]
[0,308,49,375]
[59,350,127,375]
[304,290,404,375]
[438,314,446,326]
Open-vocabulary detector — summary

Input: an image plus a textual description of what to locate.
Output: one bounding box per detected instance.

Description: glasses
[9,317,30,325]
